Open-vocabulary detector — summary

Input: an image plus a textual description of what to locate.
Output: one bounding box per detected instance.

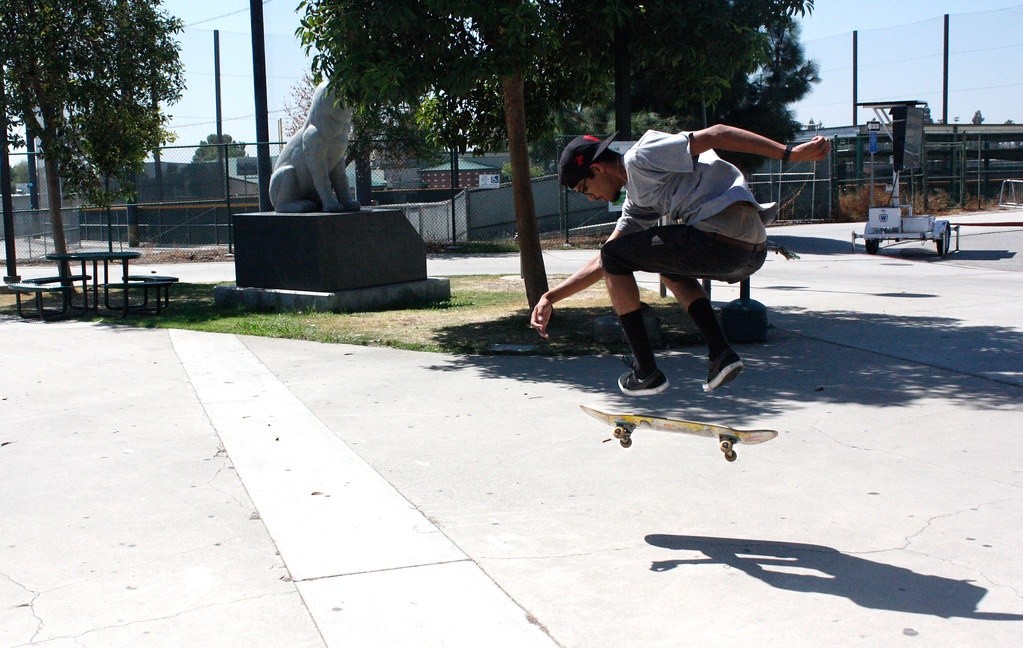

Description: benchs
[105,281,175,288]
[21,275,93,284]
[122,275,179,283]
[8,281,67,294]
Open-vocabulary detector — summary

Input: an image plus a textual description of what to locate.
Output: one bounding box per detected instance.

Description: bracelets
[783,146,792,162]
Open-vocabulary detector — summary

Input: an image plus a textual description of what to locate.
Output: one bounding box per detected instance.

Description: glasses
[582,162,593,195]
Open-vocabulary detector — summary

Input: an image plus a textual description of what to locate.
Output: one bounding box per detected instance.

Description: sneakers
[702,347,743,392]
[618,353,670,396]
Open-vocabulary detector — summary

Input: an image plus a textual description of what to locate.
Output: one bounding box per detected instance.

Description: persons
[530,124,829,396]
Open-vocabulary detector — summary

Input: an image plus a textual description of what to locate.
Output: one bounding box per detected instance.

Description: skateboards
[578,403,780,464]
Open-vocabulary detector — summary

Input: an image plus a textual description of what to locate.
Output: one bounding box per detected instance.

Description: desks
[8,250,178,320]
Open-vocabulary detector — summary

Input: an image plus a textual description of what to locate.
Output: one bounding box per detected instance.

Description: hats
[558,131,619,189]
